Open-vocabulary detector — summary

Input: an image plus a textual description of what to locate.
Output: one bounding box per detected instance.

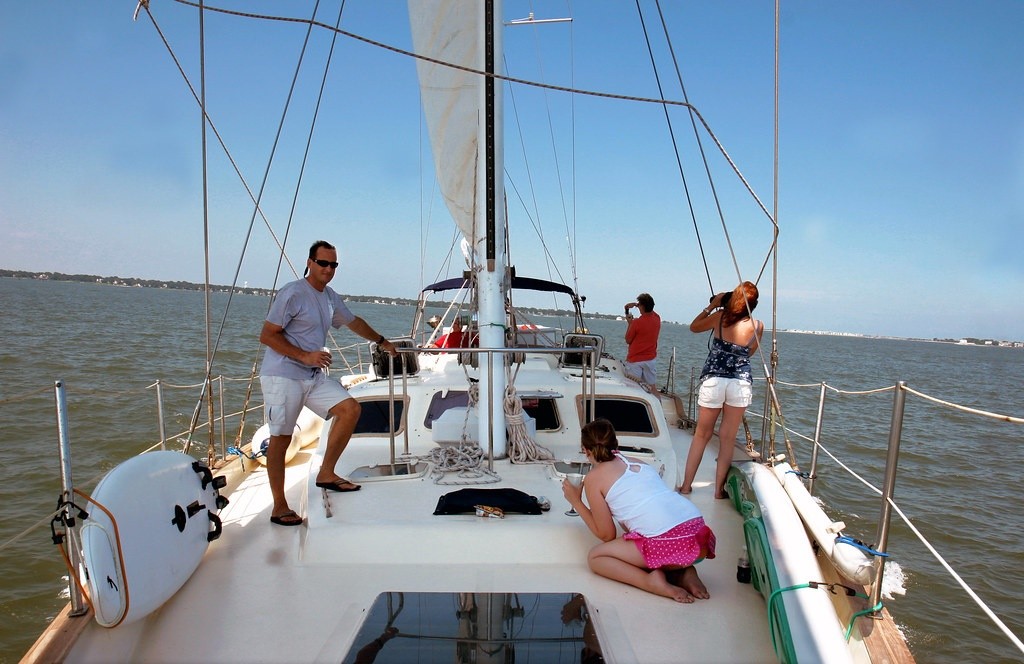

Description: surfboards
[82,450,229,628]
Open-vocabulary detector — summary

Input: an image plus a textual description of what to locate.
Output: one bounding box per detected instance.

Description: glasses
[314,259,339,269]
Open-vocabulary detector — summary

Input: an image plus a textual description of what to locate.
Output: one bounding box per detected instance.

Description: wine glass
[564,473,583,516]
[319,346,332,382]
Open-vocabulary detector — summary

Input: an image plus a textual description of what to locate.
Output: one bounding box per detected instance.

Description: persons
[561,420,715,603]
[624,293,662,402]
[430,317,478,356]
[260,242,397,526]
[677,282,764,498]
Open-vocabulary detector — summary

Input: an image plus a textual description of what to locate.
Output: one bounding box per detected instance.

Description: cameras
[626,309,629,315]
[710,291,733,307]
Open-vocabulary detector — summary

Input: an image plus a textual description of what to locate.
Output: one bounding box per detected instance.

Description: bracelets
[703,308,710,314]
[376,335,385,346]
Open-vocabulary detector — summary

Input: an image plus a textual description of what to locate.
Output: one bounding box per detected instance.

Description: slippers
[316,477,362,492]
[270,509,303,526]
